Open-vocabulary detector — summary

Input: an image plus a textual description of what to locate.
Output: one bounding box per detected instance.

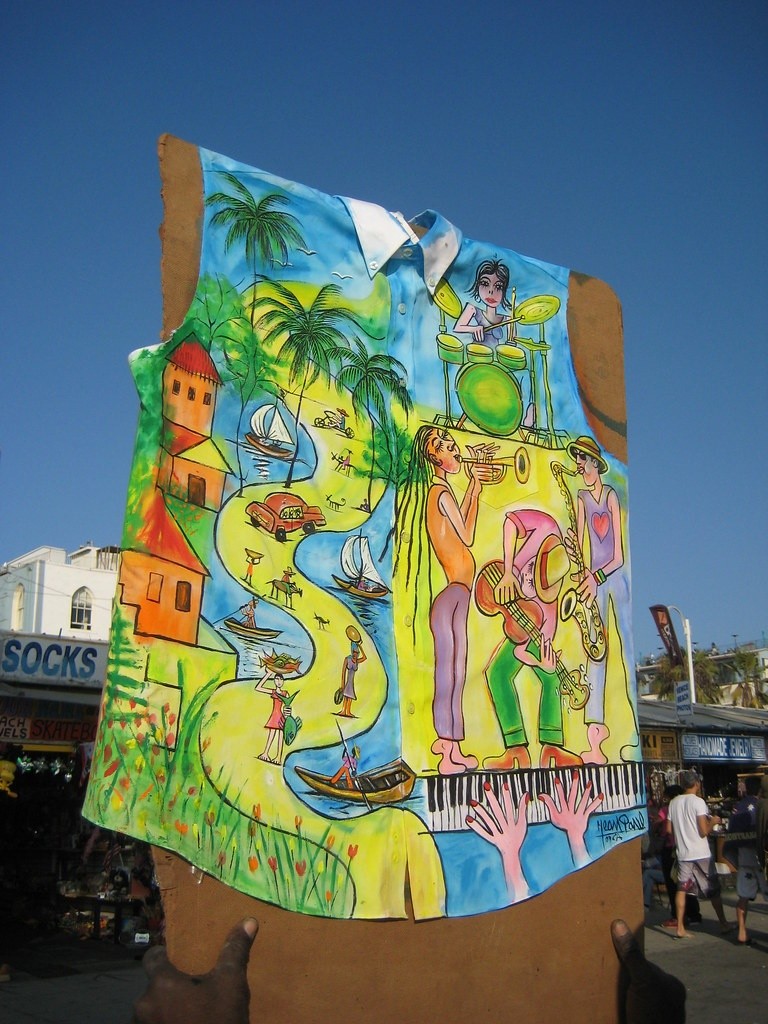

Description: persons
[643,785,692,919]
[731,768,768,943]
[666,771,738,938]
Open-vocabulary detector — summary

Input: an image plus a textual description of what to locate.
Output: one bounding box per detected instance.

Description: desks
[58,895,157,944]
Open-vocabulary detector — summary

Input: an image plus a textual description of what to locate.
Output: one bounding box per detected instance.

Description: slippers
[673,933,695,939]
[722,921,738,934]
[733,929,757,943]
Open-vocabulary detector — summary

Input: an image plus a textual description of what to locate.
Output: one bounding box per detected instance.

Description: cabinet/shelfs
[0,848,133,896]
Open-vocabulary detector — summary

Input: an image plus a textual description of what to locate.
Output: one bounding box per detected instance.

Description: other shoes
[657,918,678,928]
[0,964,16,981]
[645,905,649,912]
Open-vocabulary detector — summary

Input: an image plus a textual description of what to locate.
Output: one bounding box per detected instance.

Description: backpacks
[727,799,761,847]
